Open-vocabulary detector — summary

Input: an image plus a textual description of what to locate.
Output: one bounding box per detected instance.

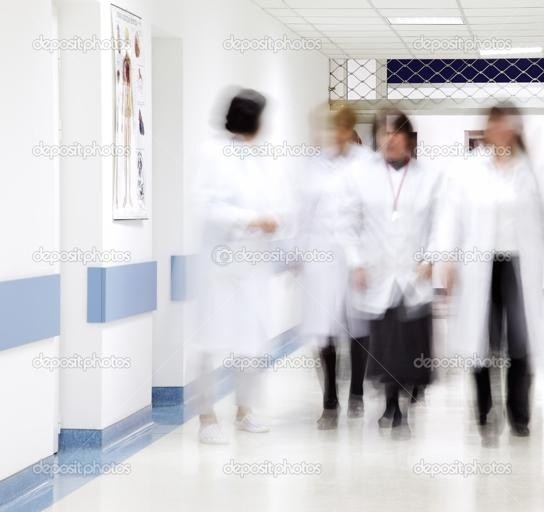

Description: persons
[288,100,375,431]
[333,106,451,430]
[438,100,543,448]
[119,25,137,211]
[184,88,293,447]
